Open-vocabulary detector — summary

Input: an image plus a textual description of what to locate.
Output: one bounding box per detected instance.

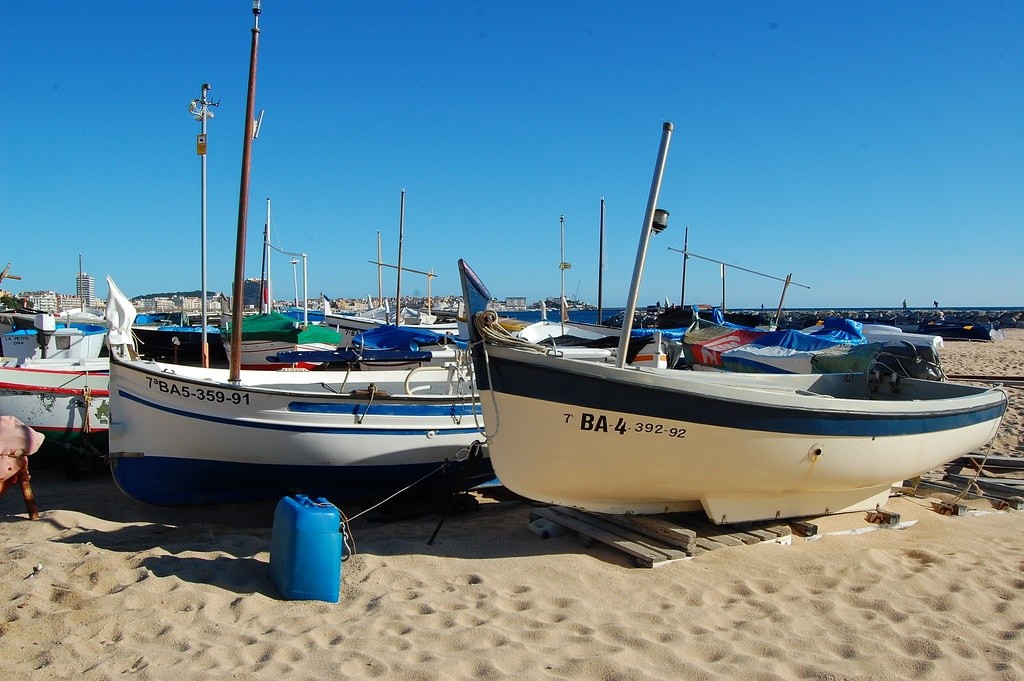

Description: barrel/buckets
[269,495,343,604]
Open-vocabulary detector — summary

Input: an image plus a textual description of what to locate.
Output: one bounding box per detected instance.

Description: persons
[902,299,907,310]
[656,301,661,309]
[933,300,939,310]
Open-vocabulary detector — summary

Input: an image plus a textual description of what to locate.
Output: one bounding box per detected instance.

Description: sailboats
[1,0,944,513]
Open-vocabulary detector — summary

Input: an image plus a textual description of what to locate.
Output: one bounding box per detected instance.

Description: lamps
[652,209,670,235]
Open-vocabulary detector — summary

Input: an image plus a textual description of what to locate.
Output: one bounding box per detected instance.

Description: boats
[456,122,1008,526]
